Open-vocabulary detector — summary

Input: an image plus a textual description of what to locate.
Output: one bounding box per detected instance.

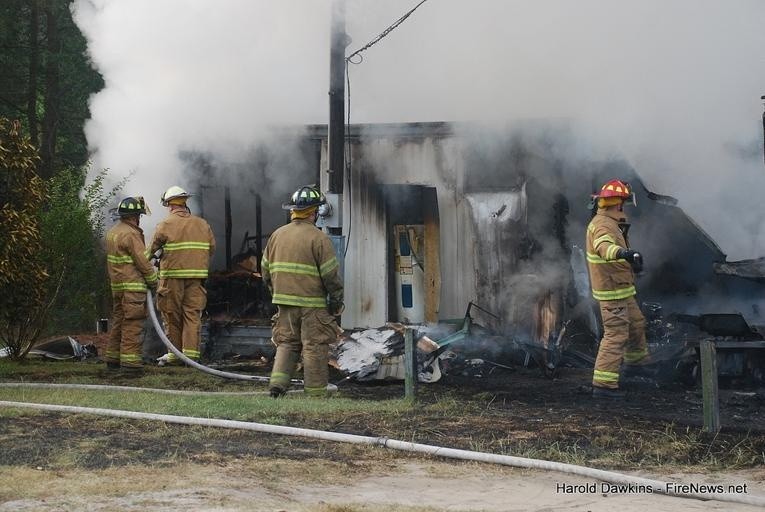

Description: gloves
[617,249,644,274]
[329,301,343,316]
[150,285,157,298]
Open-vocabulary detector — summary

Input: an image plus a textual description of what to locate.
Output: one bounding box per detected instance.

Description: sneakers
[622,364,657,377]
[326,384,338,393]
[107,362,146,377]
[592,387,627,400]
[270,386,287,397]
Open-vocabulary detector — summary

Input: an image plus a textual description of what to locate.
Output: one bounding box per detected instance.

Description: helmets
[162,186,188,206]
[591,180,630,200]
[116,197,147,215]
[281,186,326,210]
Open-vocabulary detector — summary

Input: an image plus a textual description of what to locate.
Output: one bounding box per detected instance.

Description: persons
[260,185,339,396]
[586,179,658,399]
[150,185,215,367]
[104,195,158,371]
